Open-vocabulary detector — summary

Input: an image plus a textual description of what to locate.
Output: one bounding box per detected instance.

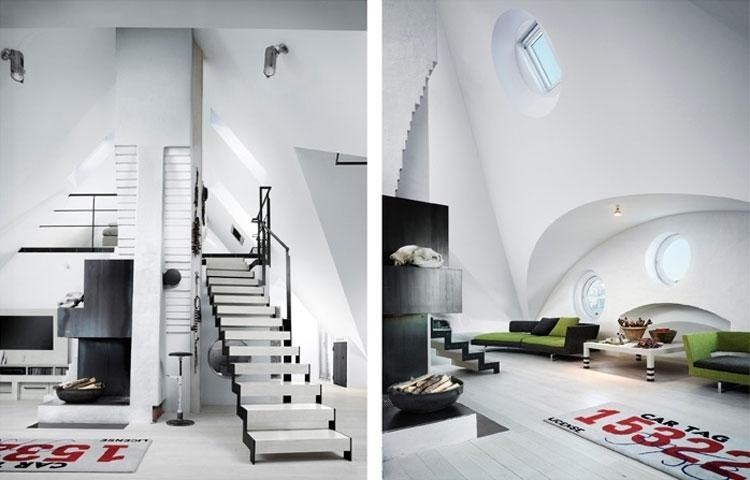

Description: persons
[388,244,445,269]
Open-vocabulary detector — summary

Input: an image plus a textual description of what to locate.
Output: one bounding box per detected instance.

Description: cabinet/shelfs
[0,364,68,402]
[57,257,132,399]
[331,338,363,389]
[381,194,465,392]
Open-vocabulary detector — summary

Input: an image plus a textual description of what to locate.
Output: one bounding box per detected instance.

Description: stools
[163,348,197,428]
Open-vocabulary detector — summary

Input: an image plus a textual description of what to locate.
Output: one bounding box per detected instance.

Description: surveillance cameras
[263,46,277,78]
[10,49,24,82]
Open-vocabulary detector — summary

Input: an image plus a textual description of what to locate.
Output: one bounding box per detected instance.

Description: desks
[579,335,697,383]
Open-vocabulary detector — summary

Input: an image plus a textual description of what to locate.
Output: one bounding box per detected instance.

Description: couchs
[469,317,602,364]
[678,329,750,396]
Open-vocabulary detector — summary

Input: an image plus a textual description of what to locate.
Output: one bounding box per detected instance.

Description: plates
[606,338,630,346]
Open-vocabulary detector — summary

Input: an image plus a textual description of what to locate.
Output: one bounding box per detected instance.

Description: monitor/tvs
[0,308,71,365]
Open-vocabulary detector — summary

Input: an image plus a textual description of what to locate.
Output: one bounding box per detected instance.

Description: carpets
[0,437,153,475]
[539,400,750,480]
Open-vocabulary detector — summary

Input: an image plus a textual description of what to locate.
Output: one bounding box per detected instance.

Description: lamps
[609,202,626,220]
[259,40,292,81]
[0,45,32,89]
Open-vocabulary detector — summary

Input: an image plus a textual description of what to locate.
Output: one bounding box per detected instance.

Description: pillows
[548,316,581,338]
[530,317,560,337]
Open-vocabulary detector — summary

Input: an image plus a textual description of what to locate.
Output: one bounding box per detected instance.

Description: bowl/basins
[56,387,104,403]
[390,376,464,411]
